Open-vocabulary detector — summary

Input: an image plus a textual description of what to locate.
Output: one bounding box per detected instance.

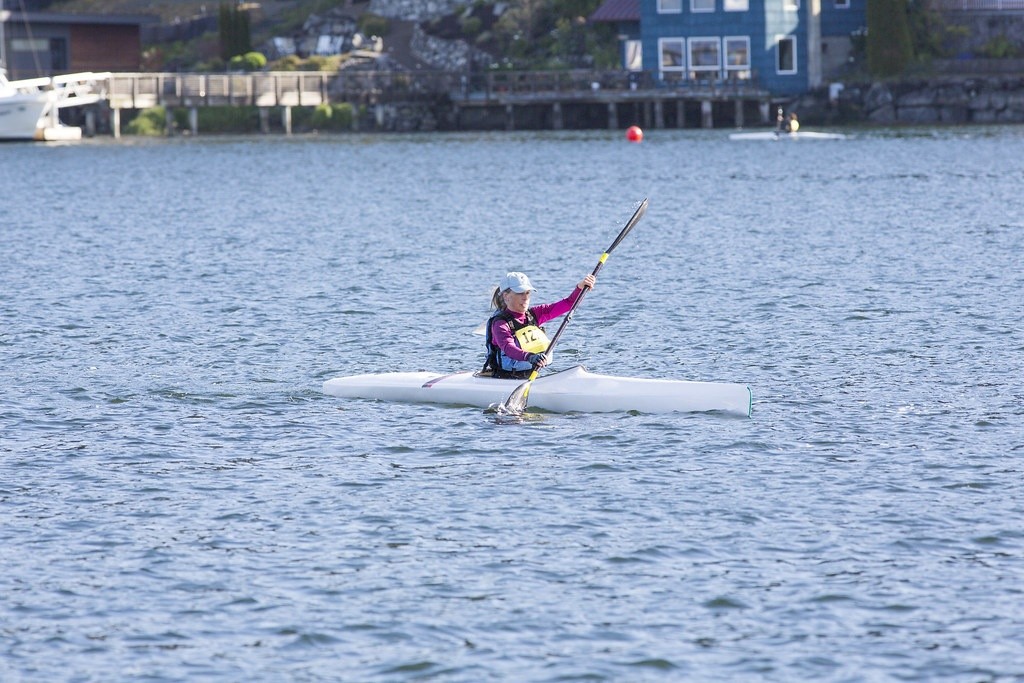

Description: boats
[324,364,753,420]
[729,131,847,140]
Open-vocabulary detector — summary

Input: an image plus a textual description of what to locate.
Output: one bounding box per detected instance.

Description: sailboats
[0,0,101,143]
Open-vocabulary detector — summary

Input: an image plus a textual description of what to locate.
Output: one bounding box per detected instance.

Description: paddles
[503,197,650,413]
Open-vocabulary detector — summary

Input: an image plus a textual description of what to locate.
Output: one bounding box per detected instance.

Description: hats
[498,272,538,296]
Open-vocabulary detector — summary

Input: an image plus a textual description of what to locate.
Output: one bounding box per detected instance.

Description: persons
[781,113,798,132]
[484,272,596,379]
